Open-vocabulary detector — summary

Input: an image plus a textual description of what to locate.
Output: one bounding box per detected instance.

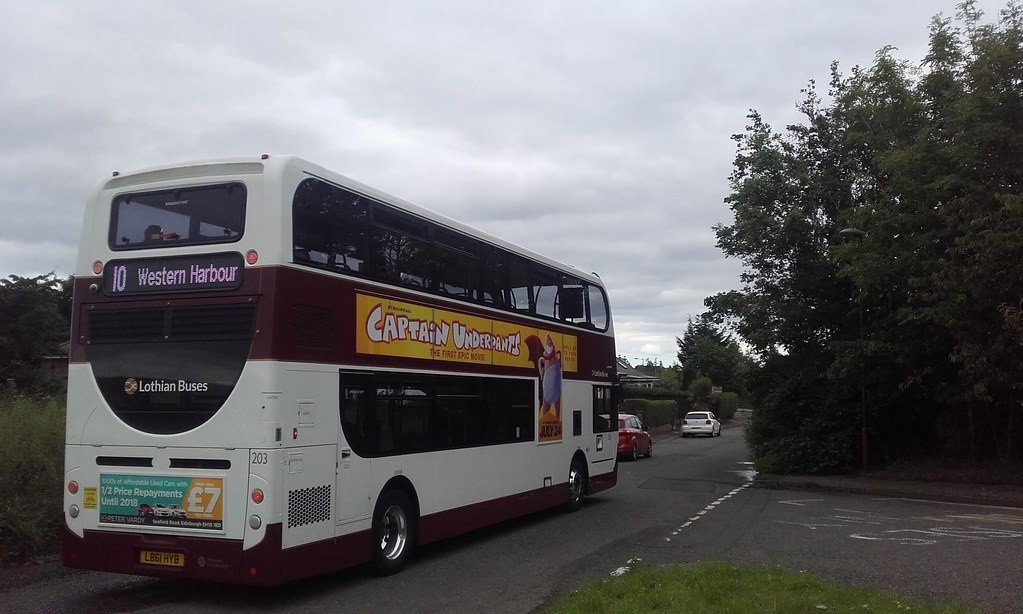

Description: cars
[598,414,652,461]
[682,411,721,438]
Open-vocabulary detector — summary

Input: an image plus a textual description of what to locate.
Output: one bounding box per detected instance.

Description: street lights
[634,358,644,365]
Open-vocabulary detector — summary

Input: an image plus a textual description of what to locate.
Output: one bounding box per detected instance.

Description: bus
[62,154,621,590]
